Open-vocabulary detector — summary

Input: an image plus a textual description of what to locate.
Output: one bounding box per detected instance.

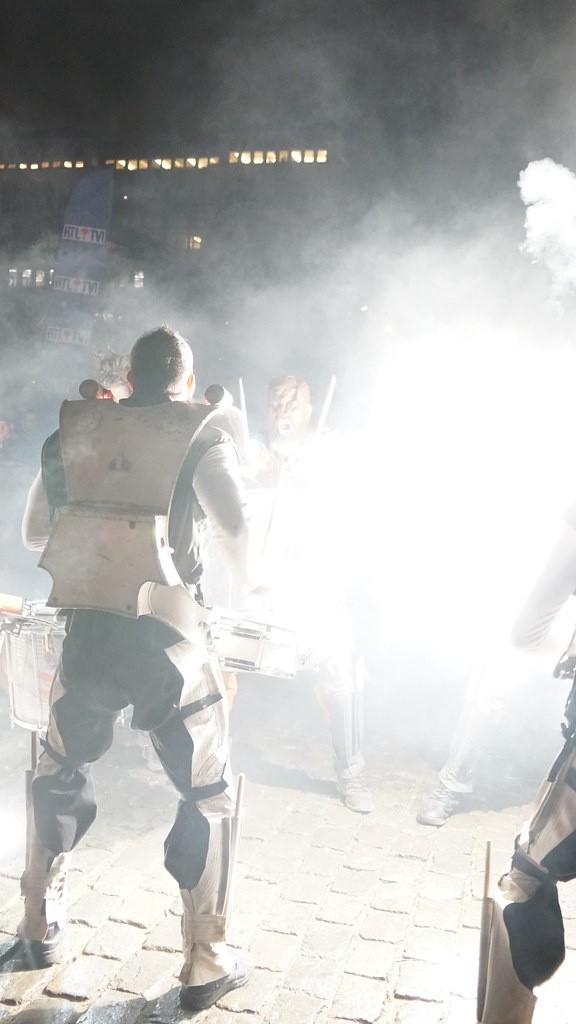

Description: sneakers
[337,776,373,812]
[417,780,459,826]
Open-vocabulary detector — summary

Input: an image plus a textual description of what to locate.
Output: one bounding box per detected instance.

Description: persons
[415,657,509,827]
[0,328,255,1012]
[477,504,576,1024]
[213,354,378,812]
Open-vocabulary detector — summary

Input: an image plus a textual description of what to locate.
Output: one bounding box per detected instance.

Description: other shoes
[180,958,254,1009]
[17,925,59,969]
